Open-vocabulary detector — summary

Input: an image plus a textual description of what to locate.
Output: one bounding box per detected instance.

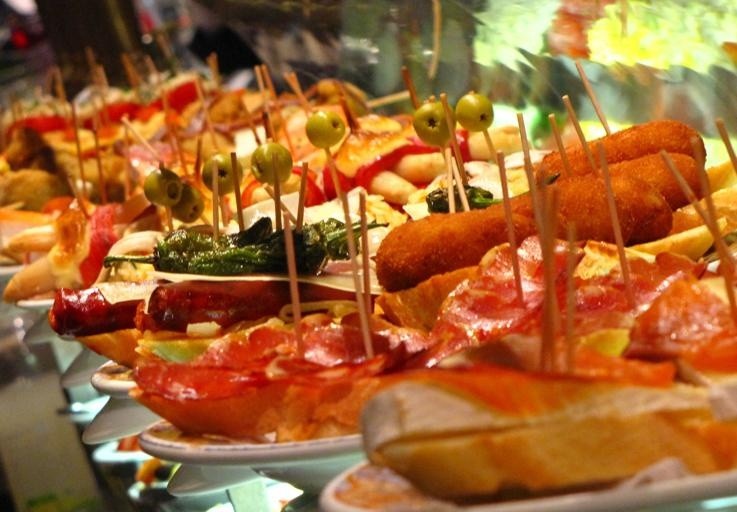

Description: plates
[0,262,736,512]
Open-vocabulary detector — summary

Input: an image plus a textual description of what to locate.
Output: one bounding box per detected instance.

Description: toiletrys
[104,216,390,276]
[425,174,560,211]
